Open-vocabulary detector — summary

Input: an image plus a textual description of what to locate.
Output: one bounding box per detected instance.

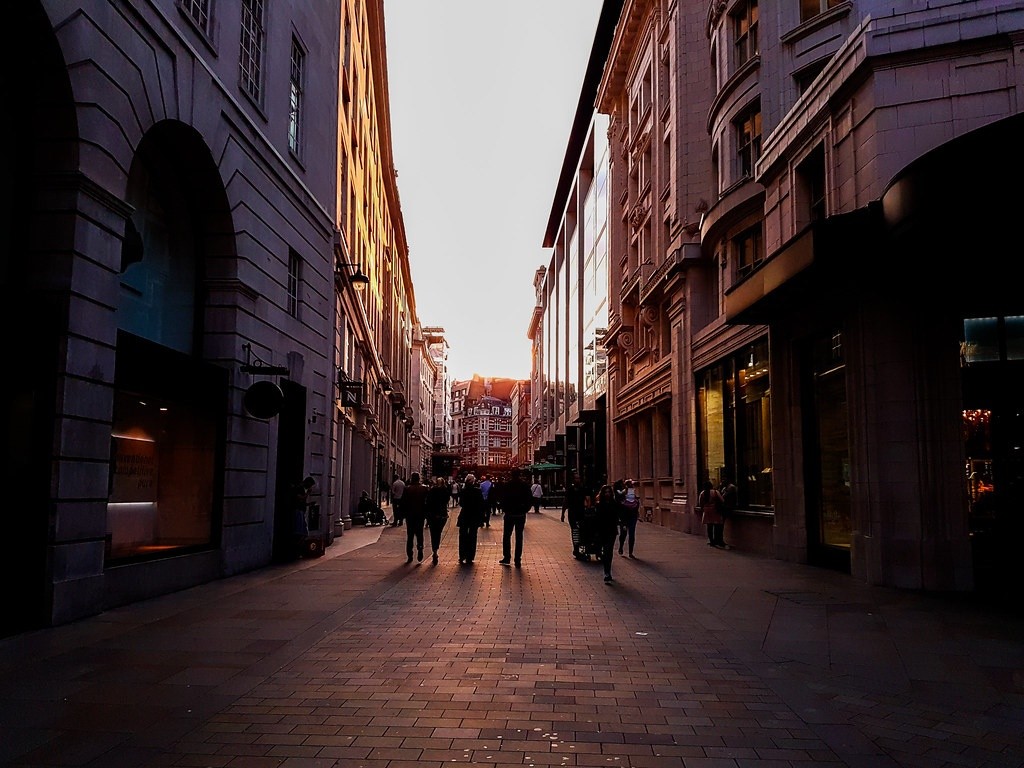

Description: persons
[593,485,619,581]
[296,477,316,537]
[456,475,485,565]
[399,473,428,564]
[427,478,451,564]
[530,479,543,514]
[698,478,737,549]
[561,470,640,560]
[358,474,504,528]
[498,466,533,567]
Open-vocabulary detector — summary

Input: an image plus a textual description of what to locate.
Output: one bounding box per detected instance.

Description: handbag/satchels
[713,489,722,516]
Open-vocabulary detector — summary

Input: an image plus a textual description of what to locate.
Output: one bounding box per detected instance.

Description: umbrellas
[518,461,566,492]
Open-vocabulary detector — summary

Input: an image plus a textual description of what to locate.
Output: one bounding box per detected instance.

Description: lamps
[334,261,369,290]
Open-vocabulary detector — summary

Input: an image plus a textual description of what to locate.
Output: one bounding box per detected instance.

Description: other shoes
[459,558,464,561]
[465,559,472,563]
[629,554,635,559]
[417,546,423,562]
[499,558,510,564]
[433,556,438,565]
[619,548,623,555]
[604,575,612,584]
[406,556,413,563]
[515,561,521,568]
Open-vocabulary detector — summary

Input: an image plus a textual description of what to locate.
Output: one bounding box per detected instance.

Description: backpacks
[488,482,496,504]
